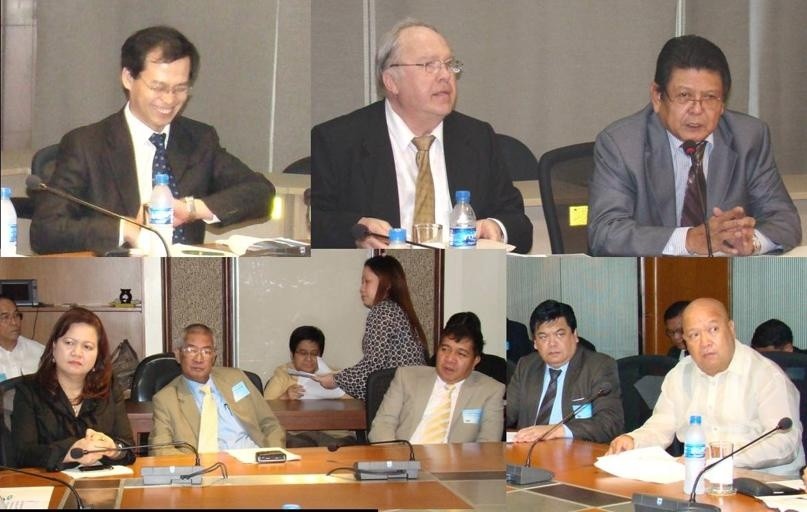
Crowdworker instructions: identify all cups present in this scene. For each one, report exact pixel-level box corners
[411,223,443,247]
[706,440,737,496]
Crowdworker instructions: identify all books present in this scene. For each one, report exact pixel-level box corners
[166,232,312,258]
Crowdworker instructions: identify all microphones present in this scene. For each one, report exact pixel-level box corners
[70,441,204,487]
[680,136,714,256]
[0,462,85,509]
[632,416,794,511]
[22,173,171,256]
[326,438,423,482]
[505,381,613,486]
[349,222,438,248]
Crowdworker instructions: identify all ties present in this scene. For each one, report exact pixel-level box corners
[149,133,184,245]
[412,136,435,244]
[198,386,218,453]
[681,141,706,226]
[536,368,562,425]
[422,384,455,444]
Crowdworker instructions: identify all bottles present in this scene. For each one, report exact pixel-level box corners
[447,189,477,247]
[1,185,18,257]
[386,228,410,249]
[148,174,174,255]
[683,415,708,494]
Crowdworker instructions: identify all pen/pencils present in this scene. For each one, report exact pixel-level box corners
[181,251,224,256]
[78,464,112,471]
[772,490,807,495]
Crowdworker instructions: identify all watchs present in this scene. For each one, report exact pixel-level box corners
[184,195,197,225]
[748,231,763,257]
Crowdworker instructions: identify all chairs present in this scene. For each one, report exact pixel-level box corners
[283,154,312,174]
[539,143,595,254]
[499,135,539,182]
[33,145,61,180]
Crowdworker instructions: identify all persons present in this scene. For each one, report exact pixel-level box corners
[602,298,807,478]
[1,298,46,383]
[508,297,627,442]
[264,325,352,403]
[367,311,507,444]
[663,298,696,358]
[10,308,136,474]
[26,22,278,257]
[304,16,533,254]
[586,32,804,257]
[145,322,288,460]
[308,255,431,401]
[750,318,802,354]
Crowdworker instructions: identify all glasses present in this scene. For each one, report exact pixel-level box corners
[390,60,463,74]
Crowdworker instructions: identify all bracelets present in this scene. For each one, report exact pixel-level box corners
[108,440,123,461]
